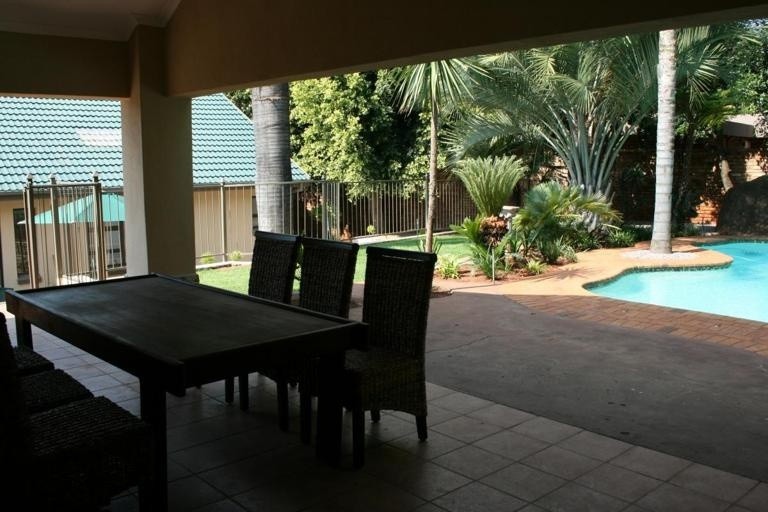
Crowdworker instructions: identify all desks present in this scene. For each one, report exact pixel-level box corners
[5,273,356,511]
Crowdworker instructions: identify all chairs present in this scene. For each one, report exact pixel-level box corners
[1,314,143,511]
[14,344,56,374]
[237,241,358,428]
[295,246,437,467]
[196,231,299,402]
[21,370,93,413]
